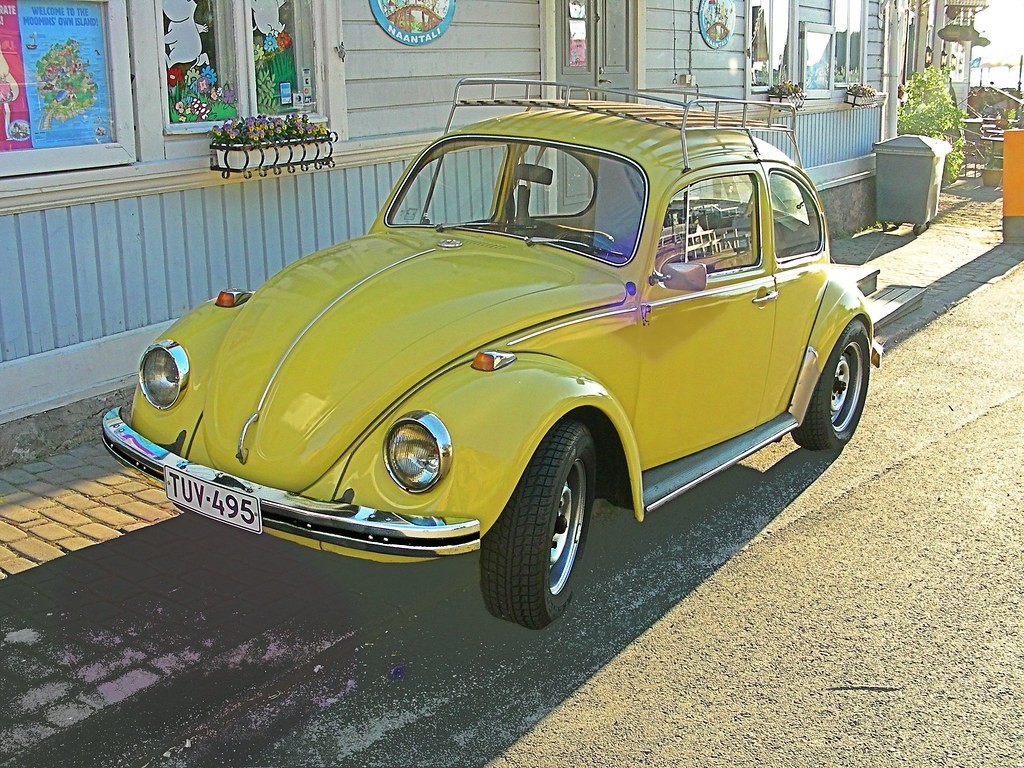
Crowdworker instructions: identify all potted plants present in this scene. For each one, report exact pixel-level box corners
[978,153,1003,188]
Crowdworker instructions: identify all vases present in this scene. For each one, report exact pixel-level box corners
[212,135,330,168]
[847,91,875,105]
[768,93,807,107]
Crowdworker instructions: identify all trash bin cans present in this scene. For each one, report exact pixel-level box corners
[872,135,954,237]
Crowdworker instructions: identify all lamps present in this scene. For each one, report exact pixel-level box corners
[925,46,934,68]
[941,50,947,67]
[950,54,957,71]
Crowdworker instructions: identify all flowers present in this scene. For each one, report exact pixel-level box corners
[848,83,878,98]
[206,112,329,148]
[767,80,803,99]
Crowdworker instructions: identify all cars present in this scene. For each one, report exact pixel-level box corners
[99,72,883,629]
